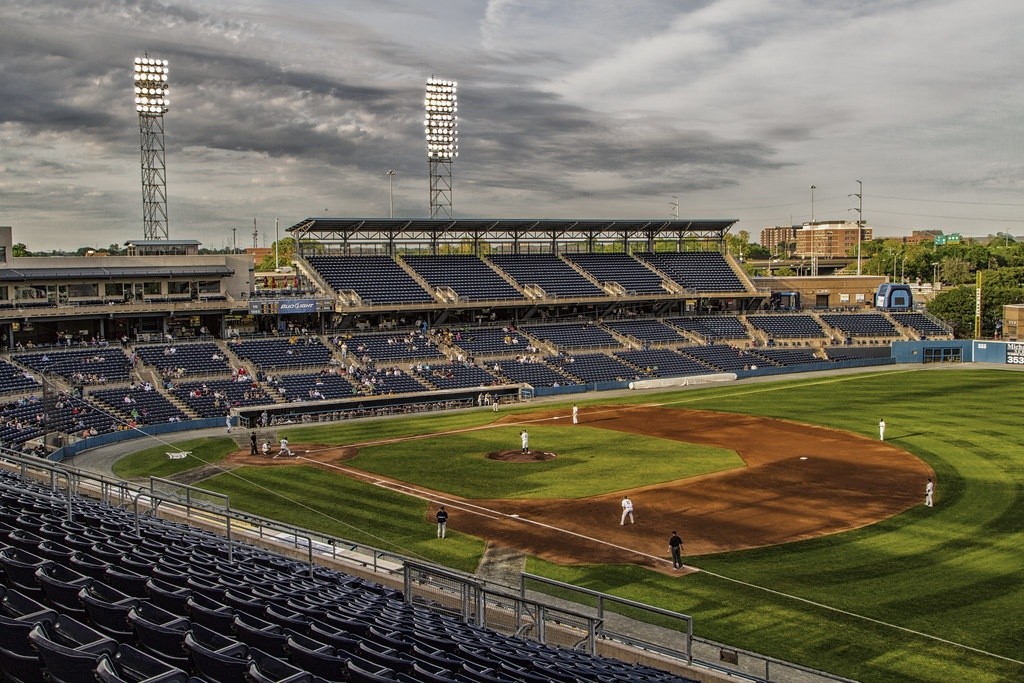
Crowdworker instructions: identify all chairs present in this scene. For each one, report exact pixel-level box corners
[0,444,693,683]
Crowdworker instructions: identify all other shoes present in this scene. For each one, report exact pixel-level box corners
[928,505,933,507]
[673,567,677,570]
[923,503,928,506]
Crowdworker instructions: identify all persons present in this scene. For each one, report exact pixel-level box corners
[262,440,271,456]
[619,496,635,526]
[696,301,775,315]
[520,430,529,454]
[0,307,659,434]
[273,437,291,459]
[250,431,259,456]
[436,505,448,539]
[263,275,298,288]
[34,426,99,457]
[879,418,886,441]
[667,531,685,570]
[682,327,1005,370]
[924,479,933,507]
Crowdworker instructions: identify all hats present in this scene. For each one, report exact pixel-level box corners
[672,531,676,534]
[441,507,444,510]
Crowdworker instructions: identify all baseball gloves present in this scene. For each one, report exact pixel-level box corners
[519,432,522,435]
